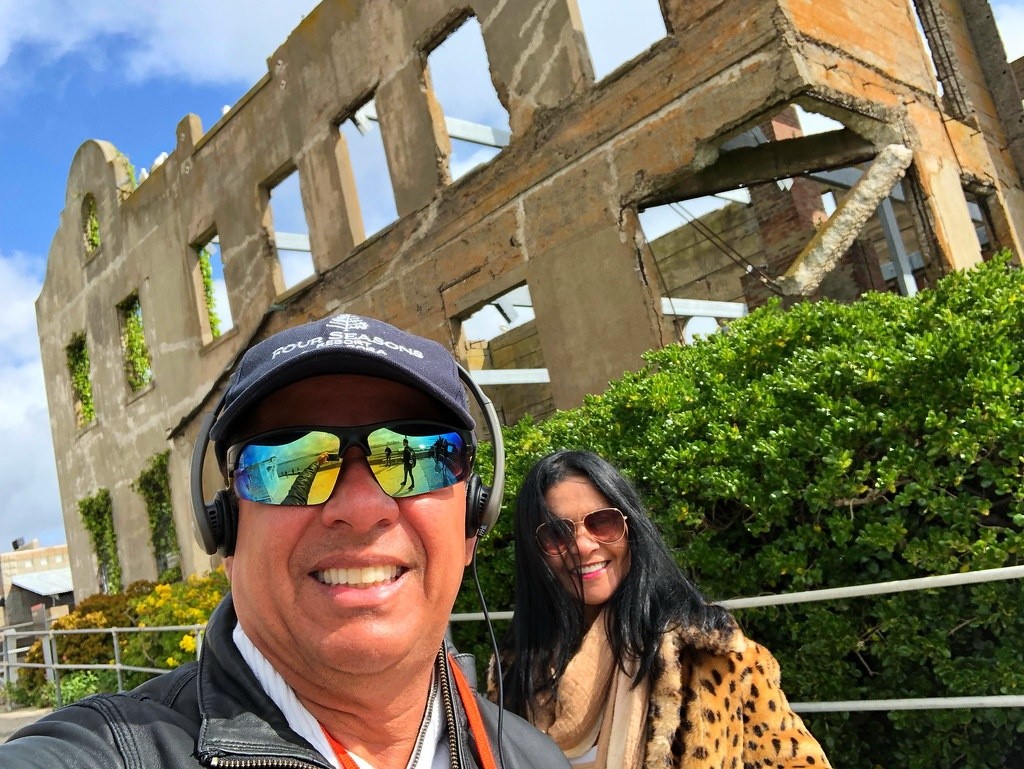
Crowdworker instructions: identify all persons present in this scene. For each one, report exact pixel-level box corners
[488,449,835,769]
[279,452,329,506]
[0,315,575,769]
[400,439,416,489]
[433,437,456,468]
[385,444,392,467]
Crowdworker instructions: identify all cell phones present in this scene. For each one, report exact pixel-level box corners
[327,453,339,461]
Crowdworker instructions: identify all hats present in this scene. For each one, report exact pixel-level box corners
[208,314,475,451]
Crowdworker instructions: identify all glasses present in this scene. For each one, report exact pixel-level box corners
[535,507,627,556]
[226,420,476,506]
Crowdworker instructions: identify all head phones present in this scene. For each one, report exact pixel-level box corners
[188,362,505,557]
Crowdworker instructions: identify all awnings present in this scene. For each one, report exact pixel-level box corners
[11,568,74,596]
